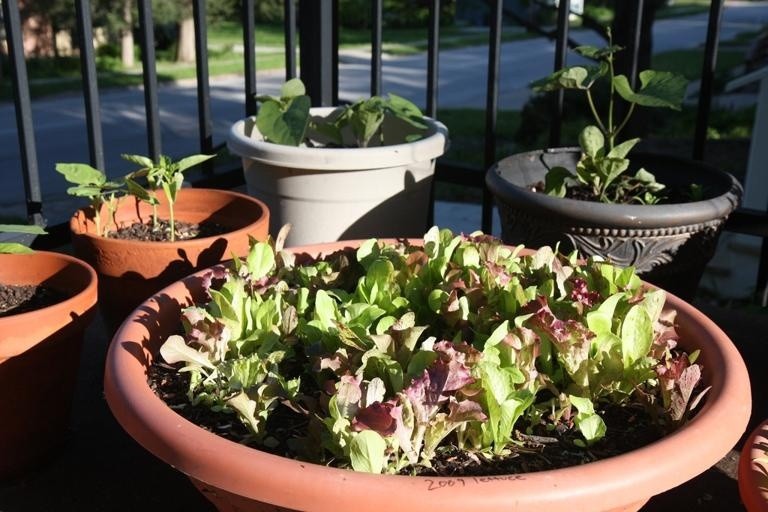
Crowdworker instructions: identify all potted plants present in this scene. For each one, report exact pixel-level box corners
[226,77,451,246]
[484,0,744,304]
[55,151,270,332]
[1,223,98,477]
[103,220,755,512]
